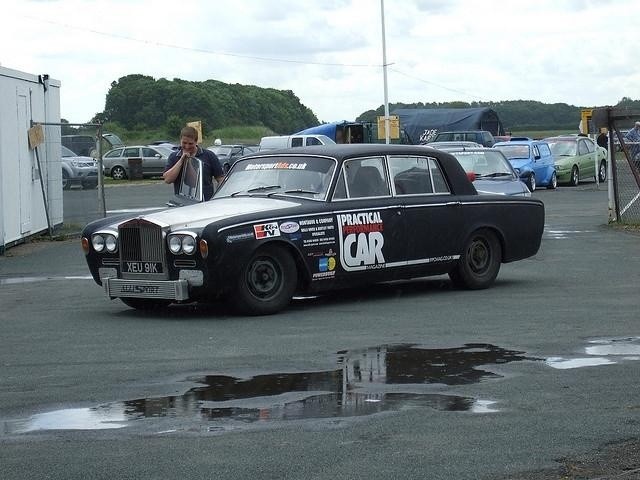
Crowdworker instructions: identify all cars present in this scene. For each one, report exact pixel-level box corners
[61,133,259,189]
[425,131,629,195]
[80,143,545,317]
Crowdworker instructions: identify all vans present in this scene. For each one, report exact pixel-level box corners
[260,134,341,149]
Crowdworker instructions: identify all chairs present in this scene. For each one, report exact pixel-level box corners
[349,165,384,196]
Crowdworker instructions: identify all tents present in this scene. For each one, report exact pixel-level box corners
[389,106,506,146]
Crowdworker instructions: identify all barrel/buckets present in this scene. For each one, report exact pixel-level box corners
[128,158,143,181]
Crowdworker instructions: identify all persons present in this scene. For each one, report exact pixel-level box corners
[162,126,225,202]
[626,121,640,176]
[596,127,609,150]
